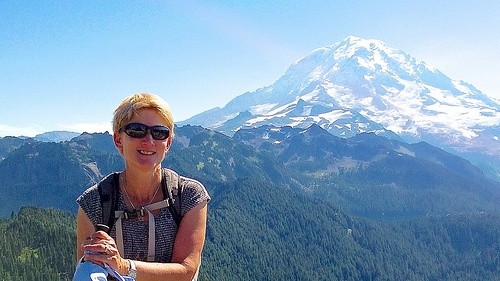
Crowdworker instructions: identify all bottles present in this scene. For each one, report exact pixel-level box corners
[73,224,110,281]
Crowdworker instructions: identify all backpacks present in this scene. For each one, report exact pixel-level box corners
[73,249,135,280]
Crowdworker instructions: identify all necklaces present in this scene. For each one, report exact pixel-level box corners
[121,173,161,221]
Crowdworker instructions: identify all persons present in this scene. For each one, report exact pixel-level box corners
[76,92,211,281]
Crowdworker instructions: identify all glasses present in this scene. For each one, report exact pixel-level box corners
[119,122,170,141]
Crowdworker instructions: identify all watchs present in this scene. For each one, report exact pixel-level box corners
[127,259,136,281]
